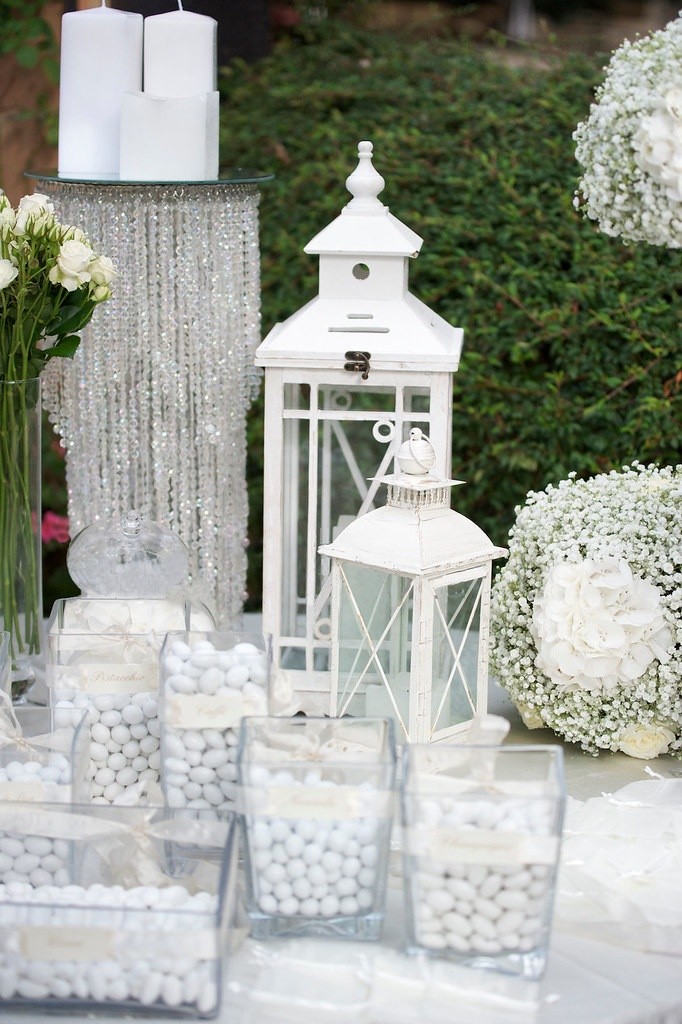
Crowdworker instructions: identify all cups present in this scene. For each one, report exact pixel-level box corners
[40,597,219,865]
[0,797,243,1024]
[1,704,94,896]
[159,630,277,881]
[397,742,565,982]
[234,714,395,940]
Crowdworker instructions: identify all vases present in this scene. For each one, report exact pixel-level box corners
[0,379,46,704]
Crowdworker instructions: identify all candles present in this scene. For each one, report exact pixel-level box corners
[143,1,217,100]
[57,1,143,181]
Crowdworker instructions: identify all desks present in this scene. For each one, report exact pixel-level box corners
[0,612,682,1022]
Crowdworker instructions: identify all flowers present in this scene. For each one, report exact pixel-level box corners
[572,7,682,250]
[480,461,682,762]
[0,184,115,675]
